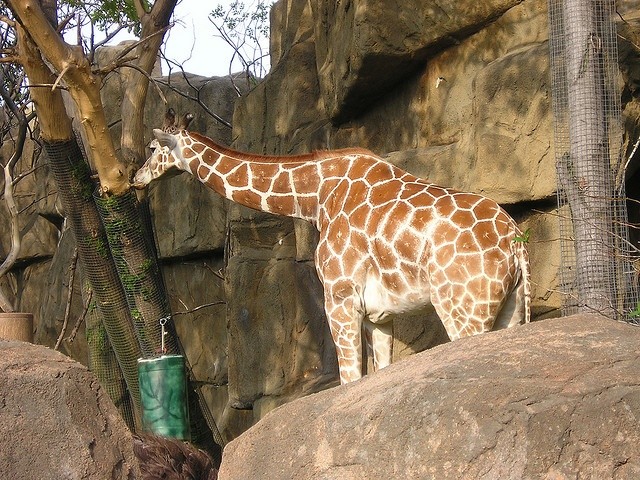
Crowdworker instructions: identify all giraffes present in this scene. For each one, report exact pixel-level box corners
[133,108,531,385]
